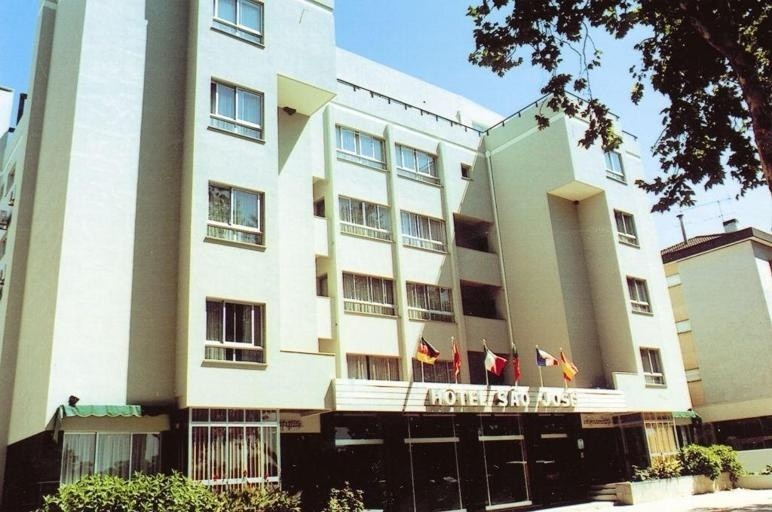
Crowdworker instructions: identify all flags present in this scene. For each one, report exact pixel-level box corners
[560,351,579,383]
[452,339,462,377]
[536,347,560,366]
[513,345,520,381]
[483,342,508,376]
[415,336,440,364]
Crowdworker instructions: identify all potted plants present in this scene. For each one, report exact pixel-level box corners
[611,441,772,506]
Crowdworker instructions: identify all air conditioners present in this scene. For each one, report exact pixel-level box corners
[0,209,8,231]
